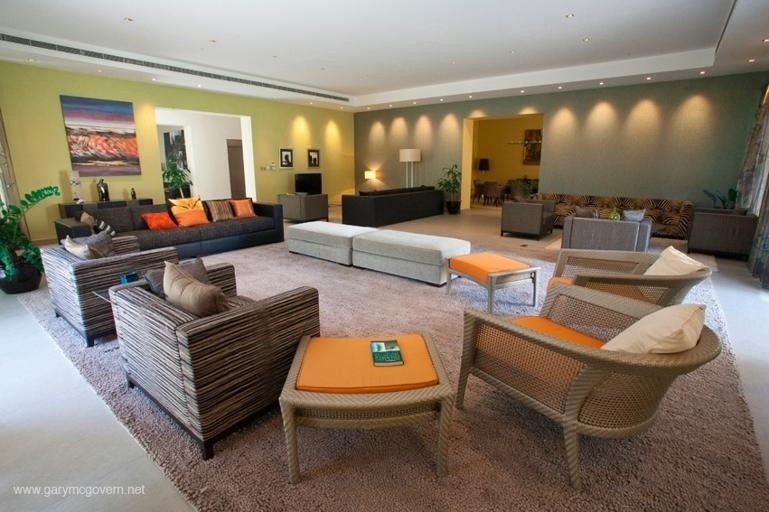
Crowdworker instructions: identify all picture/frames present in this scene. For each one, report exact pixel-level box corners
[278,147,294,169]
[306,148,320,168]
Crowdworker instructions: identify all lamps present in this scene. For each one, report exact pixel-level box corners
[398,147,422,188]
[479,157,490,199]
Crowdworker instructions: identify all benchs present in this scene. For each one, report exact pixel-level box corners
[352,229,471,284]
[287,221,381,265]
[34,165,768,493]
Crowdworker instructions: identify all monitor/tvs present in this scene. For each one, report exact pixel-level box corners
[295,173,321,195]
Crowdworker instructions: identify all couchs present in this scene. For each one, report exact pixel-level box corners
[55,198,285,259]
[342,189,444,227]
[529,192,693,236]
[501,200,557,235]
[279,192,328,221]
[688,207,757,260]
[40,236,179,347]
[108,263,320,456]
[561,213,652,252]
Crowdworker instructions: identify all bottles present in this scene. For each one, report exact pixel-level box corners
[608,207,620,220]
[131,188,136,200]
[97,179,109,201]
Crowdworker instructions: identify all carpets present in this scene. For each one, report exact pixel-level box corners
[14,239,768,511]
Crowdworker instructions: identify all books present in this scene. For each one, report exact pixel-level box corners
[369,339,404,367]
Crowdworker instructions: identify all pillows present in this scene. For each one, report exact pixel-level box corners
[163,260,232,317]
[59,235,117,259]
[599,303,705,354]
[141,199,256,229]
[622,209,644,221]
[144,257,211,301]
[643,246,710,276]
[732,207,748,216]
[73,231,116,258]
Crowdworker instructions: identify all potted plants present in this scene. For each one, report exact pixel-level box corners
[0,177,72,297]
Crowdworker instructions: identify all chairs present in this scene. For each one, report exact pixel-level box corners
[279,332,455,483]
[457,283,722,488]
[553,248,712,307]
[445,249,541,312]
